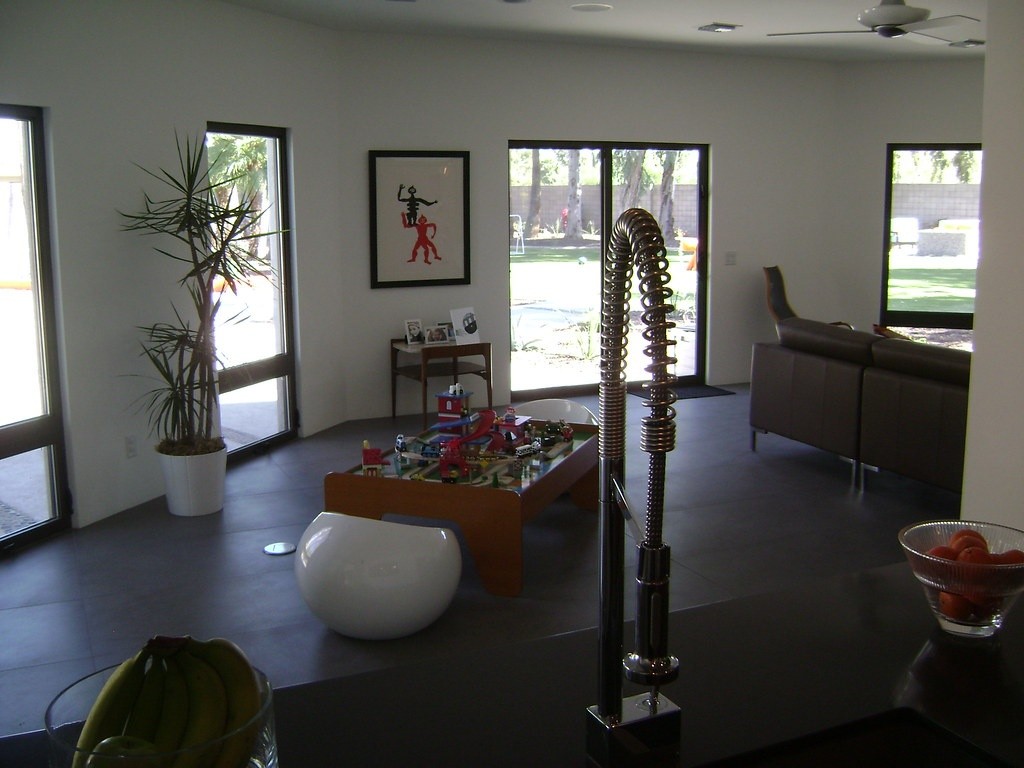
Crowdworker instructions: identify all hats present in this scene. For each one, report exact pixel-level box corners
[407,321,420,327]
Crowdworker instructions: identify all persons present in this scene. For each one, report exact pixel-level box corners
[427,328,445,341]
[406,320,426,342]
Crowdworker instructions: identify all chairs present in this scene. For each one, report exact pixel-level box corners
[748,317,972,495]
[513,399,599,425]
[294,512,460,638]
[764,266,856,330]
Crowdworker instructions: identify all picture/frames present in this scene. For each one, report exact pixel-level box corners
[368,150,470,289]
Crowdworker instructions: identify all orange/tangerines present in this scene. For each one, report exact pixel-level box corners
[924,528,1024,623]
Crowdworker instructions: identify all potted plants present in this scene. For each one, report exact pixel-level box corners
[115,125,290,517]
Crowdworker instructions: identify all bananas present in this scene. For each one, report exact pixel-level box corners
[71,634,261,768]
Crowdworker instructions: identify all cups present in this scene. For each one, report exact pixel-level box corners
[45,659,278,768]
[897,519,1024,639]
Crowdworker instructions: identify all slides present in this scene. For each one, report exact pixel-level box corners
[395,409,504,484]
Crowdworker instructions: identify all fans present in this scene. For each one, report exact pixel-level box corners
[767,0,980,45]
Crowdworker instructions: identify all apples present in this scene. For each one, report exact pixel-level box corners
[85,736,163,768]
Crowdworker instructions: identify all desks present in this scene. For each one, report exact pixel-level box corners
[324,419,600,595]
[391,339,493,431]
